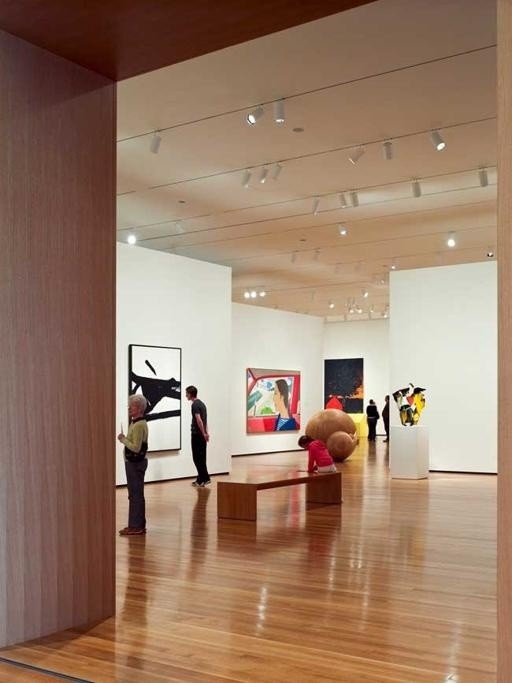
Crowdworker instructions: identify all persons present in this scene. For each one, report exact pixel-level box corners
[118,394,148,535]
[366,394,389,442]
[273,379,298,430]
[298,435,338,474]
[186,386,211,487]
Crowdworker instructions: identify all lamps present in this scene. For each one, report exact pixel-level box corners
[242,166,282,186]
[479,170,487,187]
[340,229,345,235]
[128,234,136,244]
[392,262,397,269]
[313,249,320,260]
[350,192,359,207]
[383,142,393,162]
[246,107,264,124]
[244,290,266,298]
[448,238,454,246]
[330,289,389,319]
[338,193,347,208]
[274,101,286,122]
[150,136,161,153]
[487,251,493,258]
[412,180,420,196]
[292,251,296,262]
[429,132,446,151]
[348,149,365,163]
[313,198,320,215]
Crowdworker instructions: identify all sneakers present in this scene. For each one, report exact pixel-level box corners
[118,526,147,535]
[191,481,205,487]
[204,480,211,485]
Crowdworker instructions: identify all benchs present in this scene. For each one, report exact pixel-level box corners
[216,470,343,519]
